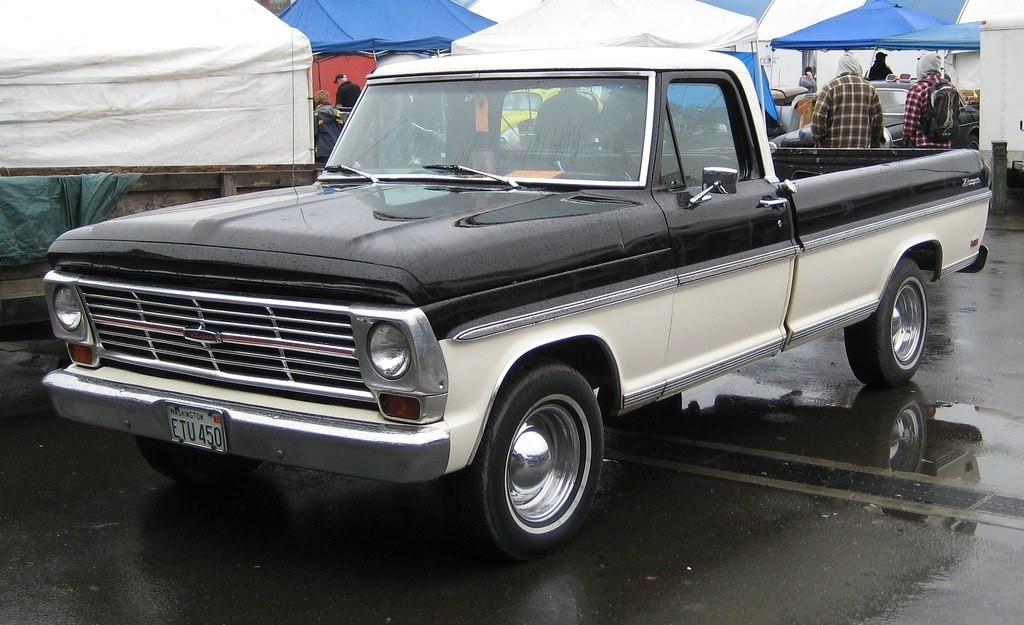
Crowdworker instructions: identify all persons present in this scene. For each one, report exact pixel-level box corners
[902,52,960,149]
[313,90,344,163]
[334,74,361,111]
[869,52,892,80]
[811,53,886,148]
[535,84,645,150]
[800,67,816,93]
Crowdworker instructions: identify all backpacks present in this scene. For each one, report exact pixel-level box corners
[915,78,960,142]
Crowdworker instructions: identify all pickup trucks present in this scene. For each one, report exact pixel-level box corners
[40,41,995,562]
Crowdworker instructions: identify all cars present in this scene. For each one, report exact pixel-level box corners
[766,73,979,148]
[499,88,603,147]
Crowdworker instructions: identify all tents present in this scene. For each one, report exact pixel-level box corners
[280,0,981,133]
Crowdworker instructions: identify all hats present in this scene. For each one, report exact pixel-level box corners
[804,67,813,72]
[334,73,347,83]
[876,52,887,59]
